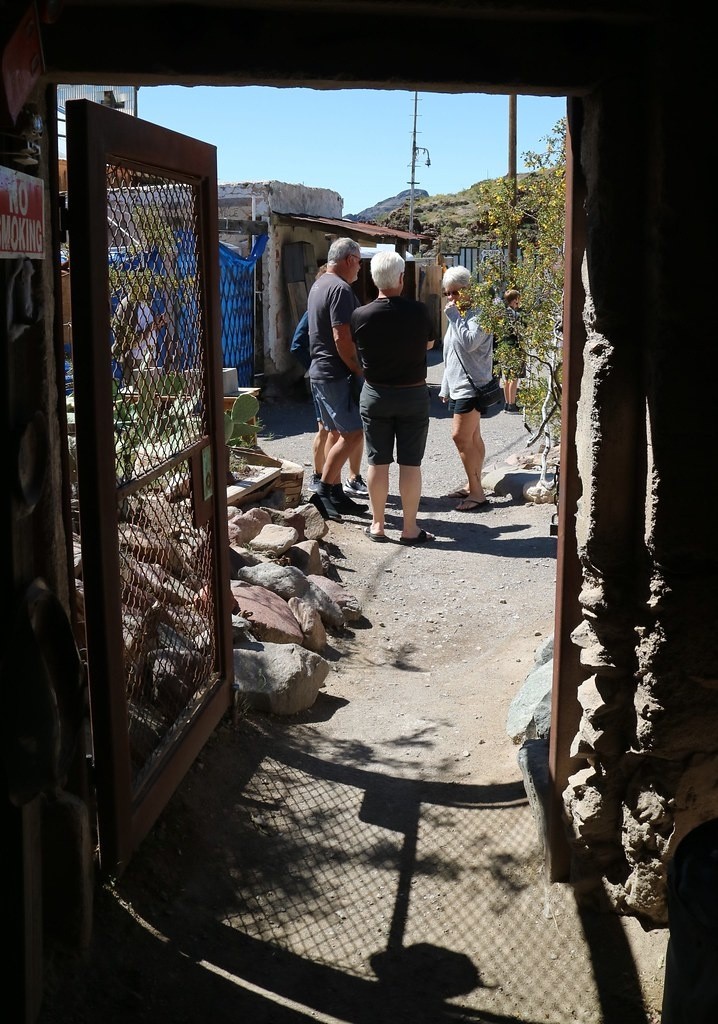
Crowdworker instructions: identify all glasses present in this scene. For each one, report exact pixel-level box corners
[346,254,364,266]
[443,287,465,296]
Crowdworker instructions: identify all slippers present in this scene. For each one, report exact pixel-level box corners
[399,530,436,544]
[456,499,489,511]
[449,491,468,498]
[362,525,387,542]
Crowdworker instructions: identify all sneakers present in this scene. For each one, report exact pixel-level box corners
[308,470,322,492]
[343,475,369,494]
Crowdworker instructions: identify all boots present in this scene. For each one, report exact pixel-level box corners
[309,482,341,522]
[329,483,368,514]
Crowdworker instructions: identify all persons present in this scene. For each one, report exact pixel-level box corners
[113,265,170,371]
[290,263,368,496]
[438,265,494,511]
[492,290,532,414]
[349,251,436,544]
[304,237,369,523]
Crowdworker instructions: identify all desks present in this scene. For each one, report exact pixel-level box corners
[116,385,261,446]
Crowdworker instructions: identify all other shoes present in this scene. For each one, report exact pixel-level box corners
[508,404,520,412]
[505,403,508,411]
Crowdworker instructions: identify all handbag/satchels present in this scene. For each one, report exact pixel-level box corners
[475,380,505,418]
